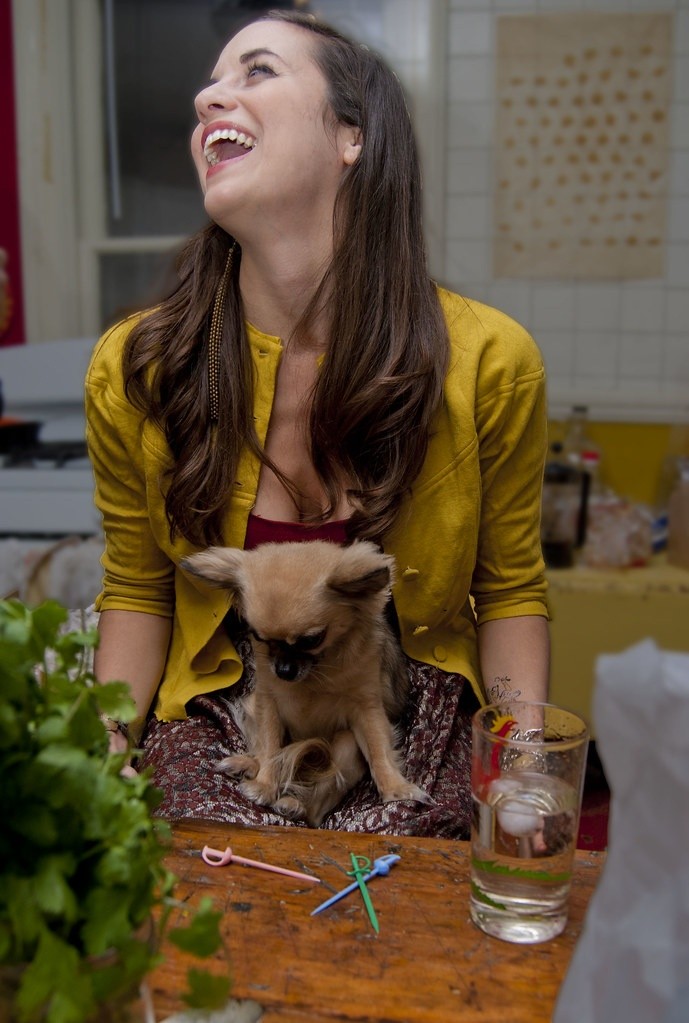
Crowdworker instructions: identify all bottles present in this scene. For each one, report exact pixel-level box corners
[583,450,617,550]
[564,406,587,470]
[668,461,689,568]
[551,443,562,466]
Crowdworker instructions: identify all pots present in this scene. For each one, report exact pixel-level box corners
[0,420,42,448]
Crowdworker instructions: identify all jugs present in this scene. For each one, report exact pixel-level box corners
[540,466,591,569]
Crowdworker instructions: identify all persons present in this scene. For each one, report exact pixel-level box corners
[86,8,552,854]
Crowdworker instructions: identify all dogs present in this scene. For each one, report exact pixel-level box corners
[185,541,437,823]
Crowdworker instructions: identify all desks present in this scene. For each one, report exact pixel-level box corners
[121,826,608,1023]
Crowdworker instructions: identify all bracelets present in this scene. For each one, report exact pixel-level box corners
[501,727,547,775]
[100,712,138,752]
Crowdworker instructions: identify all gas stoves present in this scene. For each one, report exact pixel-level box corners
[0,340,102,533]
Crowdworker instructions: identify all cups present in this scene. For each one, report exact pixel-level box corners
[471,701,590,944]
